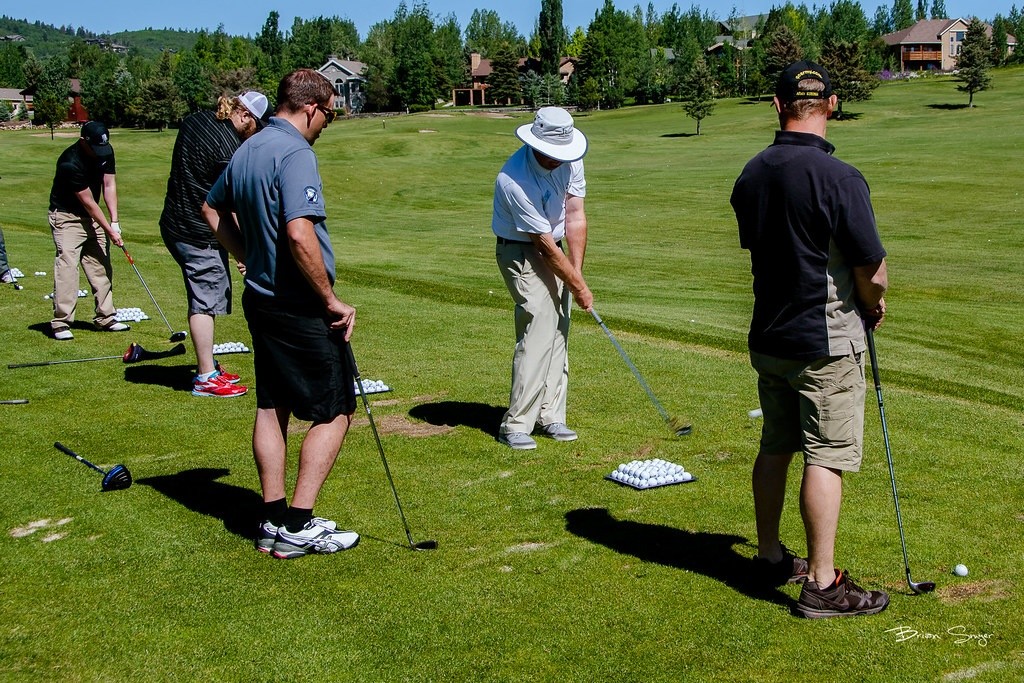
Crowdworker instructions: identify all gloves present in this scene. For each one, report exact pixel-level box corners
[111,222,121,235]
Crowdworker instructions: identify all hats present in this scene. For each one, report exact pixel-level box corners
[514,106,589,163]
[770,60,832,107]
[81,121,112,156]
[238,92,274,128]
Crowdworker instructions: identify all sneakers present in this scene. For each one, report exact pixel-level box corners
[107,321,130,331]
[1,275,18,284]
[273,519,360,559]
[256,515,337,553]
[52,326,74,340]
[533,423,578,441]
[795,569,891,619]
[191,371,248,397]
[752,540,808,585]
[191,360,240,384]
[498,432,537,450]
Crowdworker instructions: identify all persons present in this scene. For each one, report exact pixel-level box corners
[0,227,18,284]
[48,122,132,340]
[492,106,594,450]
[159,92,273,397]
[202,70,361,560]
[730,62,890,621]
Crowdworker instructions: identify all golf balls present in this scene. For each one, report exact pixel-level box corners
[955,564,968,576]
[181,330,188,336]
[354,378,390,394]
[34,271,47,276]
[10,267,24,278]
[45,295,49,299]
[19,286,23,290]
[50,289,89,298]
[611,458,692,488]
[747,409,762,420]
[114,307,148,323]
[213,341,249,356]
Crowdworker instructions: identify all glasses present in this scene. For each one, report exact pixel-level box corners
[304,101,338,123]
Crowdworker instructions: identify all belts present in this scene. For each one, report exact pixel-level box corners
[497,236,561,244]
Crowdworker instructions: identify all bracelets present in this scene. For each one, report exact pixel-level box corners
[111,220,119,223]
[863,304,880,312]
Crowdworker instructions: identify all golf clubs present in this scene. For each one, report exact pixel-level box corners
[590,307,694,437]
[52,441,132,492]
[8,355,123,369]
[865,315,937,595]
[343,327,439,551]
[1,243,20,290]
[121,245,186,342]
